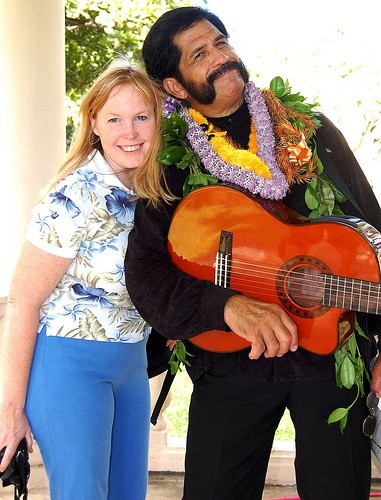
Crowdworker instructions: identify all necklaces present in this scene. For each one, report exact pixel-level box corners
[179,81,290,201]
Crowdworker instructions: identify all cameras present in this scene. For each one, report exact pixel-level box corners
[0,437,30,494]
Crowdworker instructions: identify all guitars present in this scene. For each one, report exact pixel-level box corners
[164,183,381,356]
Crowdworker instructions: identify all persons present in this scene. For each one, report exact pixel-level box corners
[122,7,381,500]
[0,51,179,500]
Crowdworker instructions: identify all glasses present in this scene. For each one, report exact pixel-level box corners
[363,392,381,450]
